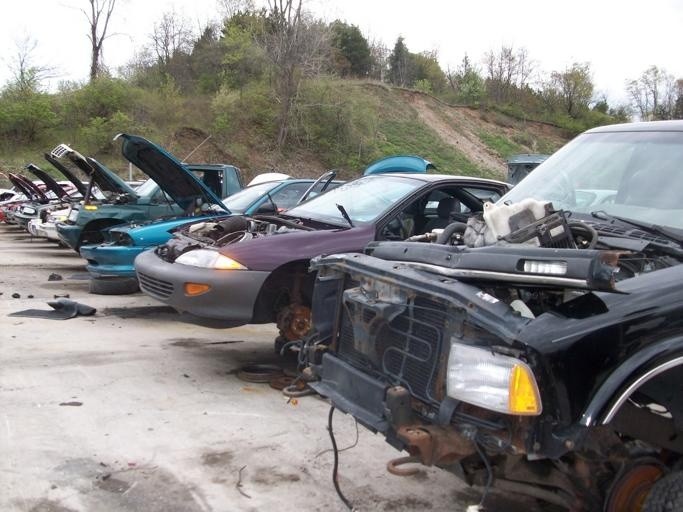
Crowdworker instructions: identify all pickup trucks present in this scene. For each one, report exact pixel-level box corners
[55,157,245,252]
[301,119,683,512]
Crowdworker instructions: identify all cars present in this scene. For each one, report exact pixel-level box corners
[79,132,347,278]
[134,171,515,343]
[0,143,106,240]
